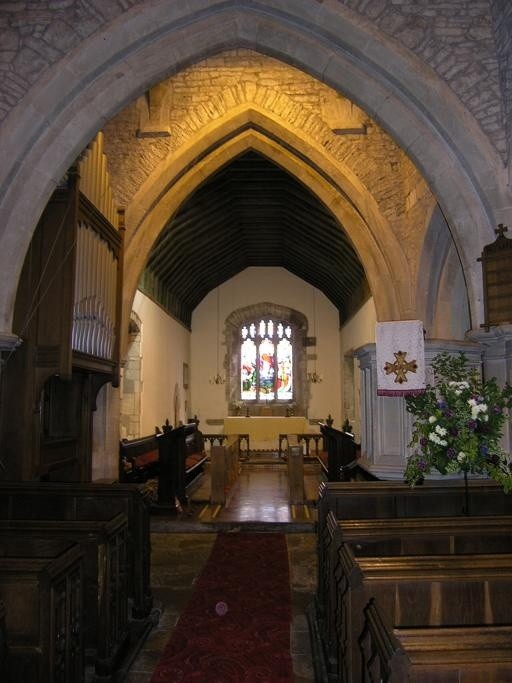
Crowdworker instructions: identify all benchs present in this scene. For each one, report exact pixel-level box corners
[0,478,163,683]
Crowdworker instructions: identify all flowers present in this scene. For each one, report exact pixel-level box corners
[403,350,511,497]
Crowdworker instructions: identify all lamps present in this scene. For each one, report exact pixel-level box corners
[376,321,426,396]
